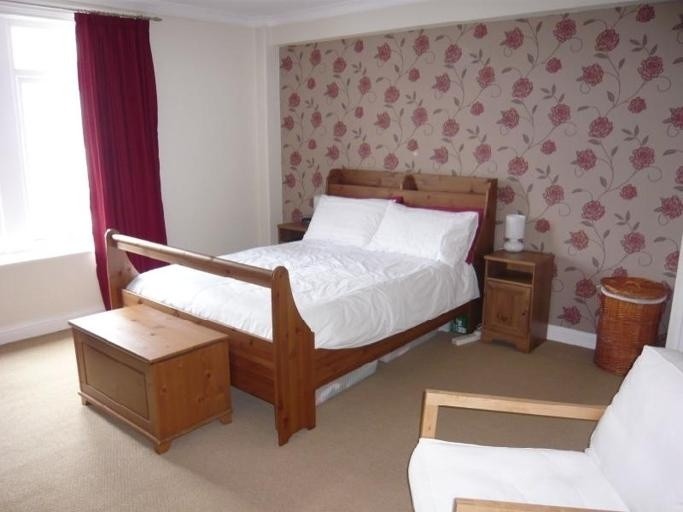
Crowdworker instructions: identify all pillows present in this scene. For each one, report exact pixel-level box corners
[370,202,478,265]
[303,195,392,249]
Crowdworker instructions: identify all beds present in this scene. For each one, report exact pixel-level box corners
[105,165,496,446]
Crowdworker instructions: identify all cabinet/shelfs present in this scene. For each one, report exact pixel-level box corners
[67,303,233,455]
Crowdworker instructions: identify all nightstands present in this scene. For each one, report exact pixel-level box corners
[276,222,306,244]
[479,248,556,353]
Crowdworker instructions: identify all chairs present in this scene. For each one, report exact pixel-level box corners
[407,346,683,512]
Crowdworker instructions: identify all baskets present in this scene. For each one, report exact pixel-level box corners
[594,275,673,377]
